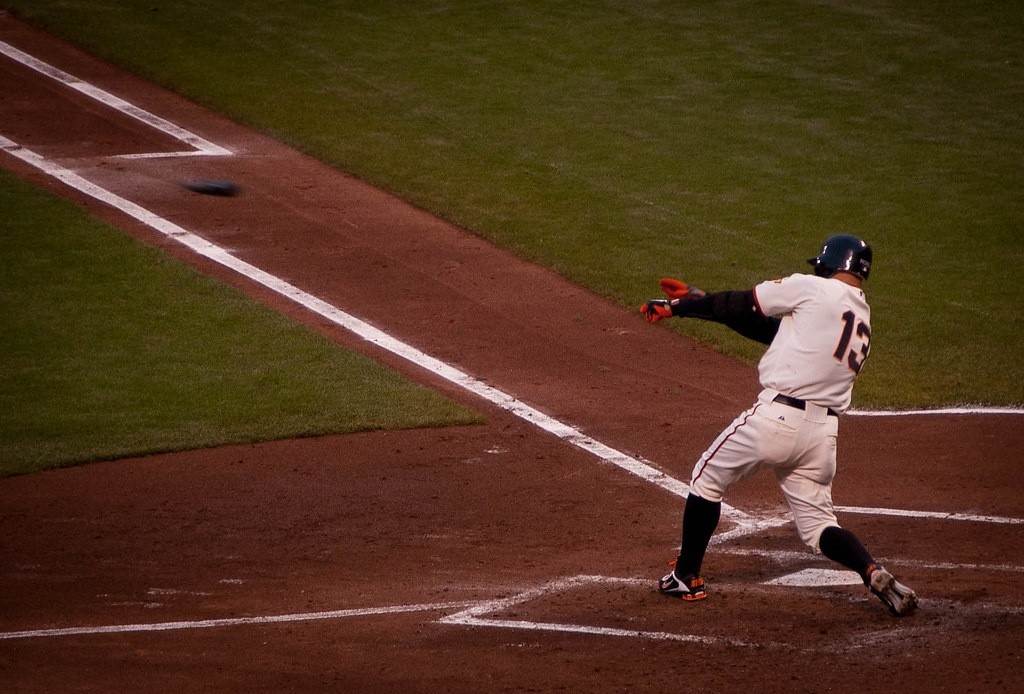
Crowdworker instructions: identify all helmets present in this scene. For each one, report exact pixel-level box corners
[807,234,872,282]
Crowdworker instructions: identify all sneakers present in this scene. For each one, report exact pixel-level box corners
[656,570,706,600]
[863,564,920,615]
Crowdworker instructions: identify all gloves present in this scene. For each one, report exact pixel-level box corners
[660,279,706,301]
[639,299,673,324]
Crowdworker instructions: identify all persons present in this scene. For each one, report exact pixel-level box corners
[639,235,919,611]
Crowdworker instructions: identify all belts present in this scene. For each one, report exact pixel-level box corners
[773,394,838,417]
